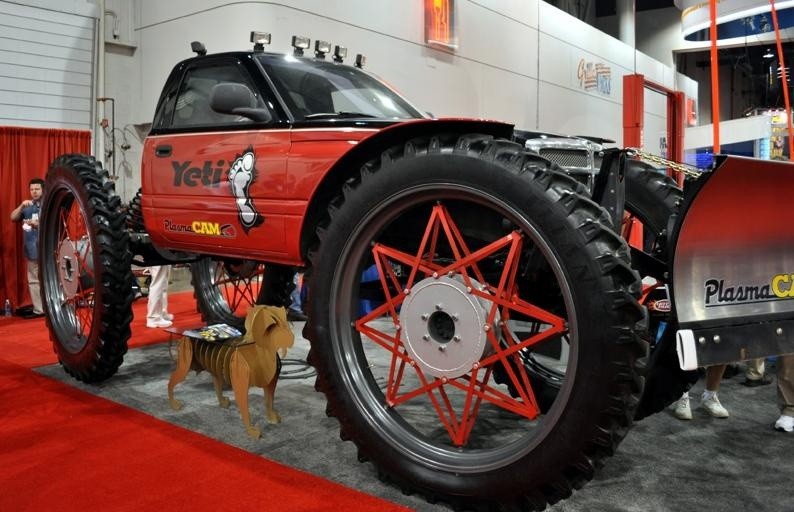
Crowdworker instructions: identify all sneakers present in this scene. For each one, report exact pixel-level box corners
[774,415,794,431]
[674,396,692,420]
[698,390,729,418]
[146,313,174,328]
[287,312,308,321]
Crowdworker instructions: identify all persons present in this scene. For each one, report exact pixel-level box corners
[147,265,175,328]
[10,178,46,320]
[774,356,794,434]
[674,363,730,420]
[724,357,772,388]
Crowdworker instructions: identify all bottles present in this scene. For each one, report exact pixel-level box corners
[32,203,39,222]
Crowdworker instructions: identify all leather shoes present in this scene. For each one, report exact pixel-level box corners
[745,374,774,387]
[24,312,45,318]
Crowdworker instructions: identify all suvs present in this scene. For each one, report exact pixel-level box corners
[36,32,794,512]
